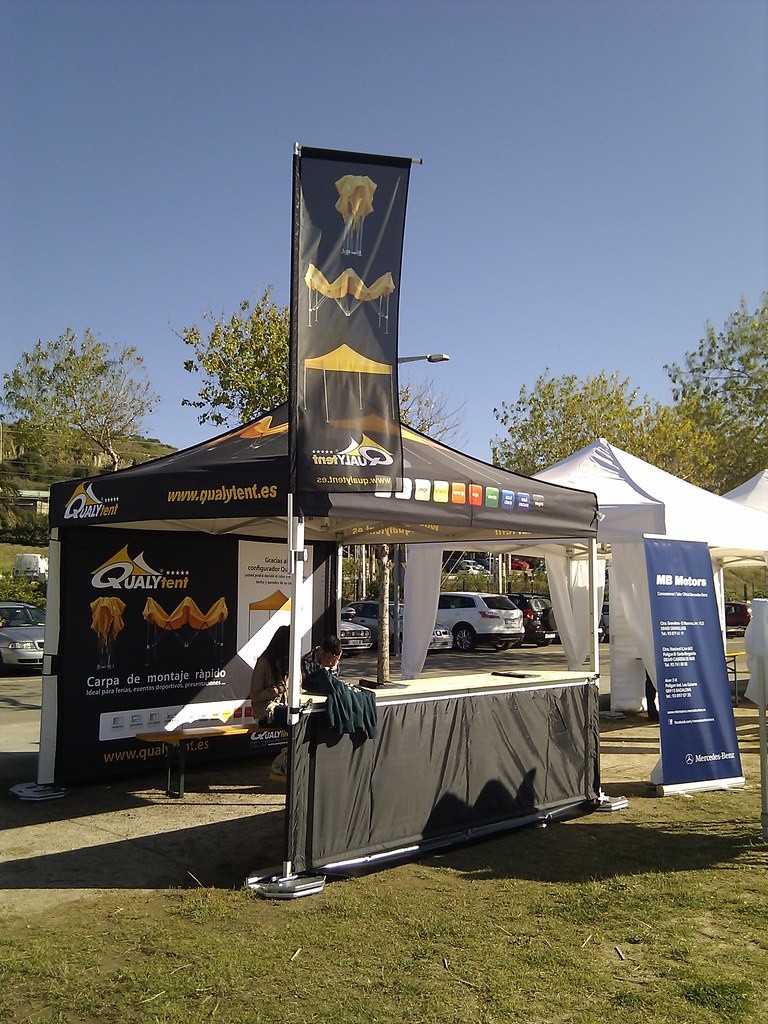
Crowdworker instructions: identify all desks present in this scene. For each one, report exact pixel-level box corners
[636,651,746,708]
[300,669,599,878]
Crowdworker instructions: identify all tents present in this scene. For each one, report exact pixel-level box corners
[9,373,629,899]
[400,436,768,838]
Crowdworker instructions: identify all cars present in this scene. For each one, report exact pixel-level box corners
[596,602,613,643]
[340,620,376,657]
[443,555,546,575]
[0,601,47,674]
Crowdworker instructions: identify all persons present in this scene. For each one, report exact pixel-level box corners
[299,636,343,682]
[250,625,301,730]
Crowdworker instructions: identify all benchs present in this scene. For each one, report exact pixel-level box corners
[141,725,271,798]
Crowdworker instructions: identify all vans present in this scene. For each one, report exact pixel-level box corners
[12,552,48,585]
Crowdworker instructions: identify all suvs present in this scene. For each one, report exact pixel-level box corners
[342,598,454,657]
[499,592,559,646]
[435,591,526,653]
[725,601,752,638]
